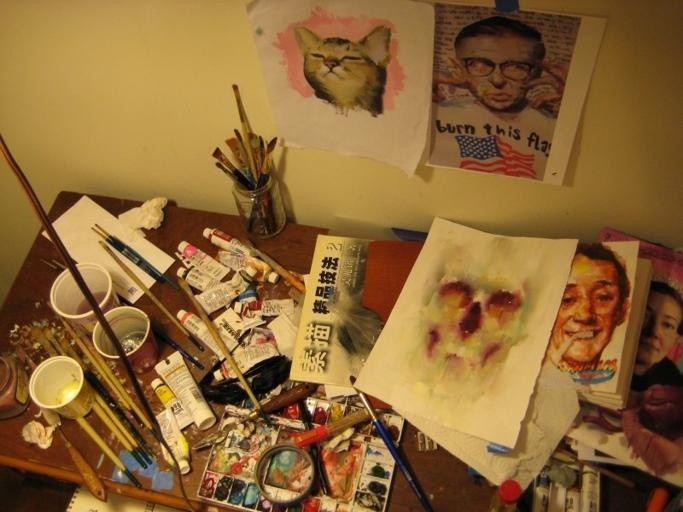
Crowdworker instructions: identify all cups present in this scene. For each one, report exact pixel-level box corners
[26,356,95,420]
[231,175,288,239]
[51,261,161,374]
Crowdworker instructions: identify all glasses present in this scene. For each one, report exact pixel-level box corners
[462,56,533,81]
[199,331,291,403]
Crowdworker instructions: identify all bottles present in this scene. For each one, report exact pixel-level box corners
[0,356,30,419]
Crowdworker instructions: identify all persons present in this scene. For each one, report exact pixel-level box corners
[630,280,683,392]
[431,17,567,182]
[553,242,631,381]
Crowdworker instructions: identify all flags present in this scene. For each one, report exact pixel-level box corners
[455,135,537,179]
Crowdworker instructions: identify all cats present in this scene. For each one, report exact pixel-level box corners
[293,25,391,117]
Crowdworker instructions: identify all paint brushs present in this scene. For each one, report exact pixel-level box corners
[206,85,287,187]
[349,375,438,511]
[178,277,274,427]
[4,322,165,488]
[84,223,205,355]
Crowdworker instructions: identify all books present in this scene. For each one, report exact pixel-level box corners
[543,240,651,411]
[532,460,600,512]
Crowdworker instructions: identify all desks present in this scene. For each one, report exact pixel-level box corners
[0,190,682,510]
